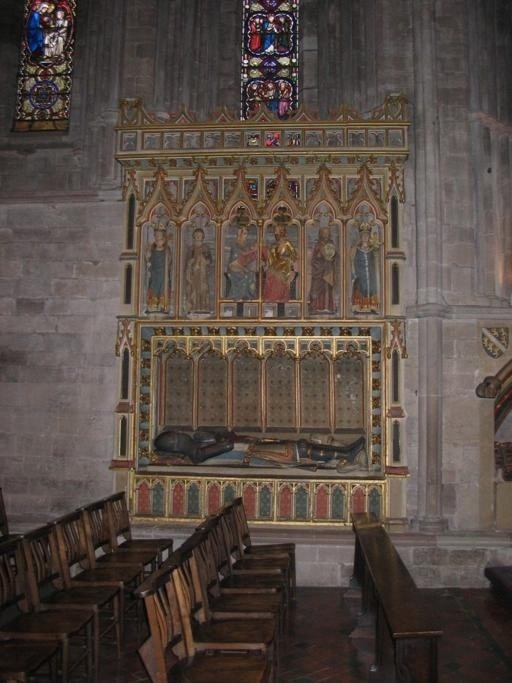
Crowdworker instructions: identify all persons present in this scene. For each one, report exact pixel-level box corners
[185,228,212,312]
[153,427,366,467]
[348,220,382,315]
[309,227,337,314]
[146,230,171,315]
[262,226,299,302]
[225,227,257,300]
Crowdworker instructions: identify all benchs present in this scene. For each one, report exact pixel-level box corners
[350,512,444,683]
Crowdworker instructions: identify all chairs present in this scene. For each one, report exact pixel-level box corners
[133,497,295,683]
[3,493,174,683]
